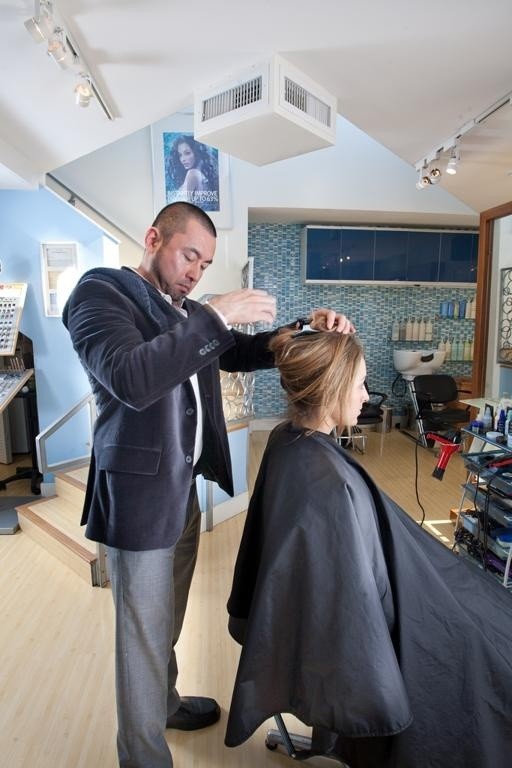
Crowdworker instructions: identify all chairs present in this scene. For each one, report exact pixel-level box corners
[337,380,392,454]
[399,373,473,451]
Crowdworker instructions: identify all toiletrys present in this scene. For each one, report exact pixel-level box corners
[392,315,432,340]
[441,293,476,318]
[437,337,474,360]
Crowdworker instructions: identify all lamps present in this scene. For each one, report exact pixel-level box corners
[18,1,102,117]
[408,135,465,195]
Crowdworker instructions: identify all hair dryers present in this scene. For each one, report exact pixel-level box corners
[424,430,462,481]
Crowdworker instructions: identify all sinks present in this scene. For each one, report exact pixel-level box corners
[392,349,446,381]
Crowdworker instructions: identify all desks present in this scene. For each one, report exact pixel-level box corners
[457,396,501,458]
[1,368,44,495]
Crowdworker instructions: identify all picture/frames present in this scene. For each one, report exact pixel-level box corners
[149,110,237,232]
[36,237,84,320]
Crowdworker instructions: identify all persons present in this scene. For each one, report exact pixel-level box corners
[62,201,358,768]
[168,135,219,210]
[267,321,370,446]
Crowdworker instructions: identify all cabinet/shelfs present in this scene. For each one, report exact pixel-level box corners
[371,227,440,287]
[296,224,374,288]
[389,315,476,365]
[436,230,479,289]
[448,421,512,593]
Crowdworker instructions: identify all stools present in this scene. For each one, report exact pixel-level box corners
[262,705,351,768]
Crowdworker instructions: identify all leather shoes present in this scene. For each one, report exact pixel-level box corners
[166,695,221,732]
